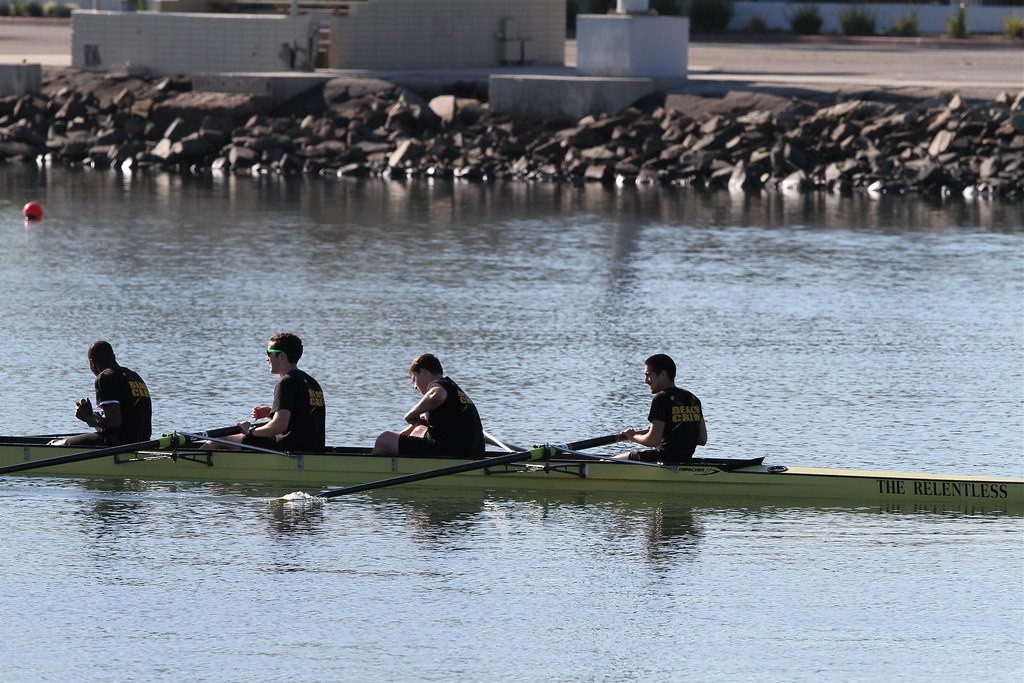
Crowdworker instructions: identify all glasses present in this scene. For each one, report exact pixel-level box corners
[266,349,287,357]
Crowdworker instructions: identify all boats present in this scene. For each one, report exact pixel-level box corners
[1,432,1024,513]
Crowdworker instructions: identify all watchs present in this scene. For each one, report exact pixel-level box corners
[248,426,257,436]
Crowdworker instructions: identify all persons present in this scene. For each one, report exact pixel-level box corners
[610,354,708,463]
[199,332,325,450]
[372,353,485,459]
[46,341,151,445]
